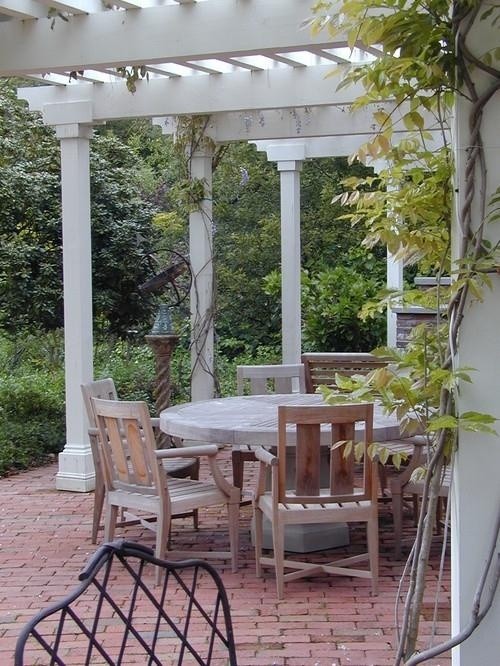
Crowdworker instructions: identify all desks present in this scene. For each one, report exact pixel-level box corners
[157,390,437,555]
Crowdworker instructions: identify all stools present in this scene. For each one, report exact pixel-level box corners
[395,430,456,556]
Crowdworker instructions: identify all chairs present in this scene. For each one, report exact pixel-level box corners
[297,351,425,530]
[233,363,312,503]
[15,538,238,666]
[90,398,242,587]
[77,378,203,551]
[251,405,383,600]
